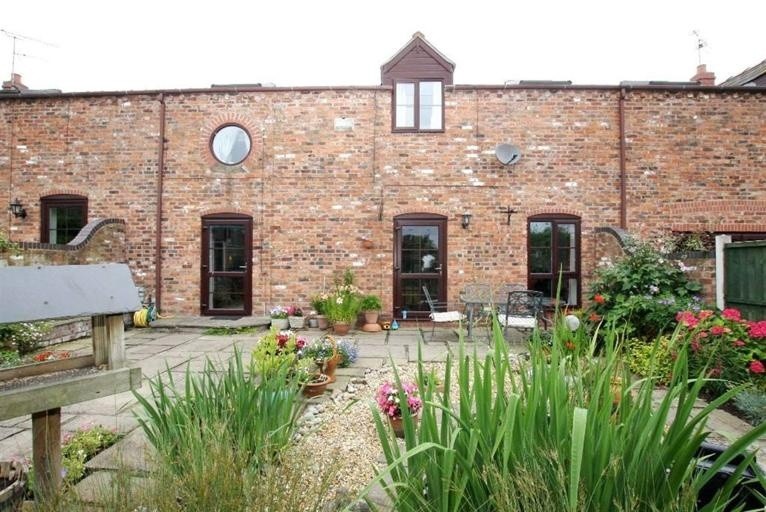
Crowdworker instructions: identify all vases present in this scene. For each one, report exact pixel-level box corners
[389,413,419,438]
[297,373,330,398]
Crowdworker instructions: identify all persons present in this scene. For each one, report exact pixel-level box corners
[422,255,437,299]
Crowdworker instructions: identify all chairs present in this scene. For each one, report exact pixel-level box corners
[422,282,566,342]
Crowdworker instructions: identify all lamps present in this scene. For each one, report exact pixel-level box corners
[462,211,473,229]
[9,197,27,220]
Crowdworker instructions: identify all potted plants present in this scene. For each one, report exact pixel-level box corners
[360,293,383,332]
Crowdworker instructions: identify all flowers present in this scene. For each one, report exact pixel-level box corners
[254,267,364,385]
[373,380,423,420]
